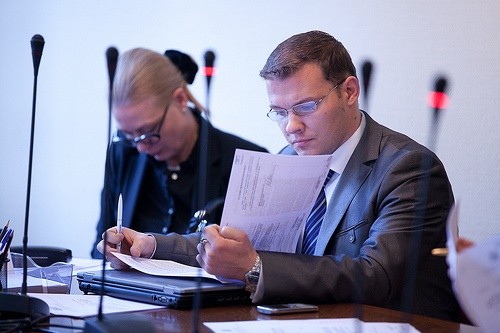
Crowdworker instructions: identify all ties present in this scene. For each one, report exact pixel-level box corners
[302,169,335,255]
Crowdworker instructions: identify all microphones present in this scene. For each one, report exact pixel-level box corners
[192,49,217,333]
[83,47,154,333]
[353,59,373,333]
[21,34,46,294]
[401,76,449,333]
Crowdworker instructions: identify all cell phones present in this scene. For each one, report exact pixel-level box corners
[256,303,318,315]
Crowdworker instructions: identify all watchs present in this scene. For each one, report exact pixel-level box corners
[245,253,262,294]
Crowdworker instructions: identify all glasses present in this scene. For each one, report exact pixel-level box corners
[267,75,357,122]
[117,87,178,147]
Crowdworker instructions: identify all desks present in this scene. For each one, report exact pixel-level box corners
[0,258,484,333]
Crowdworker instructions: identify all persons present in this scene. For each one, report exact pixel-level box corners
[97,30,476,326]
[90,46,271,259]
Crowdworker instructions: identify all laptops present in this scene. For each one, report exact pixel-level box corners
[77,267,254,307]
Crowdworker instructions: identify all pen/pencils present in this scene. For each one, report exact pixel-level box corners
[0,219,14,272]
[116,192,124,253]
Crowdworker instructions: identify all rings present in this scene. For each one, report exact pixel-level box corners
[200,239,207,250]
